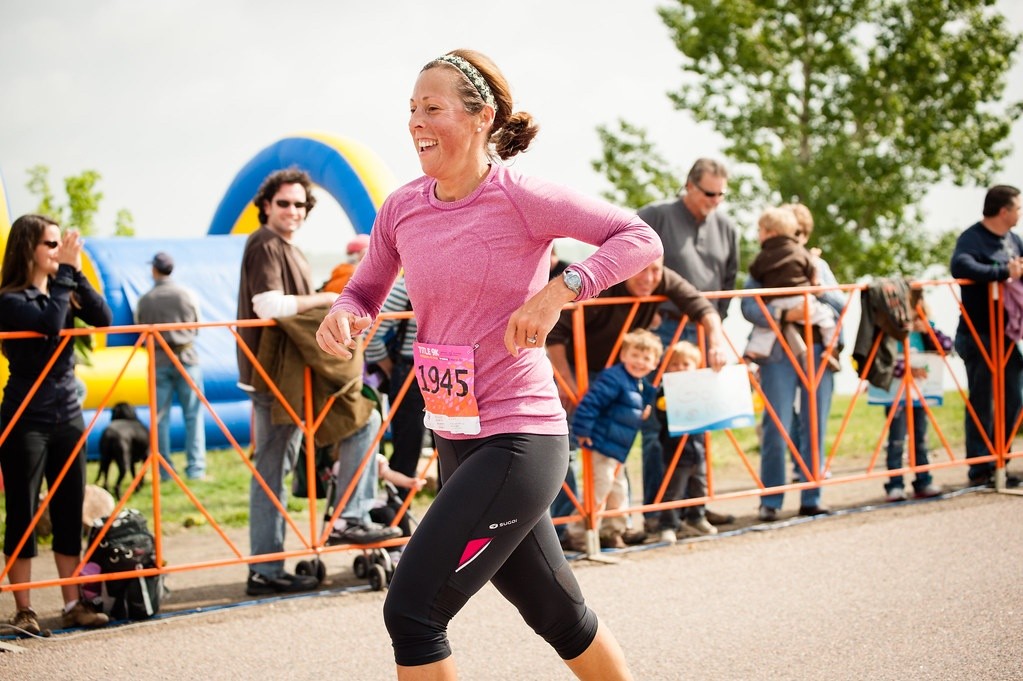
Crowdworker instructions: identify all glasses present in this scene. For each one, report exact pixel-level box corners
[275,200,308,209]
[695,183,726,198]
[795,230,805,237]
[39,240,59,249]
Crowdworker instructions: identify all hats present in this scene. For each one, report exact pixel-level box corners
[346,234,370,253]
[147,252,174,273]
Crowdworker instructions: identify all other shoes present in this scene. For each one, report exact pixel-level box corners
[758,504,780,520]
[686,517,718,535]
[739,356,760,390]
[820,347,841,371]
[644,517,660,532]
[792,473,801,482]
[885,487,908,501]
[799,504,832,516]
[705,506,735,525]
[659,527,677,544]
[969,470,1022,488]
[600,531,625,548]
[914,483,942,498]
[190,474,214,482]
[819,469,832,478]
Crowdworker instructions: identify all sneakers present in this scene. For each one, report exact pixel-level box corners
[328,518,403,545]
[14,608,52,638]
[60,601,109,629]
[245,569,319,596]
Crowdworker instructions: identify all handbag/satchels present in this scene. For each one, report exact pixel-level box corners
[367,301,413,376]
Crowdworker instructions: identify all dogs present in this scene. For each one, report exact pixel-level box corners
[93,401,150,502]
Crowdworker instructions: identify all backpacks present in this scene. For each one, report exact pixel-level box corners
[79,507,171,621]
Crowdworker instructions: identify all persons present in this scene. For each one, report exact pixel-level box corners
[138,251,215,481]
[314,48,663,681]
[950,186,1023,489]
[0,216,112,637]
[234,167,850,598]
[882,284,952,501]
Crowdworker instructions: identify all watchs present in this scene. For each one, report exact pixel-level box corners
[562,270,581,294]
